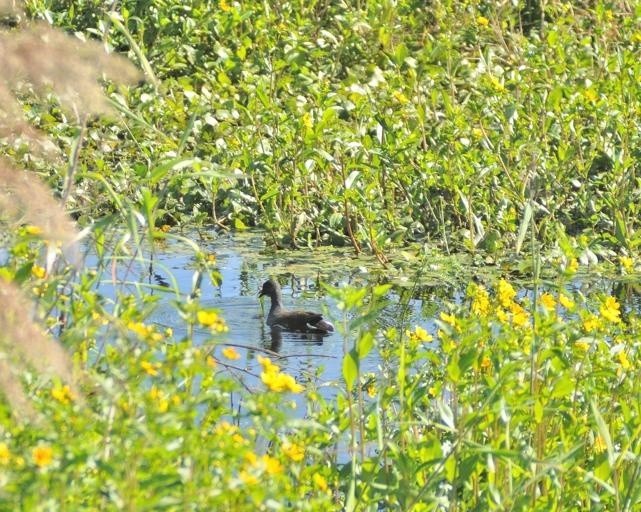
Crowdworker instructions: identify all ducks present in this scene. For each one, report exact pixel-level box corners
[257,279,334,333]
